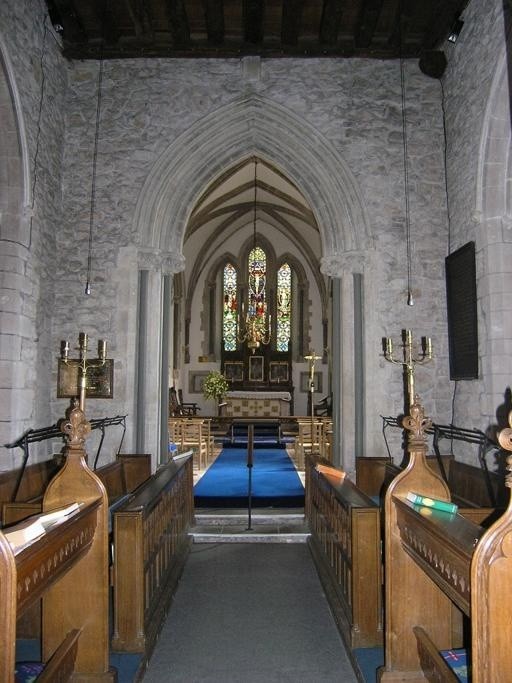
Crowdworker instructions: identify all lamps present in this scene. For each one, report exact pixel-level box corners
[235,157,275,356]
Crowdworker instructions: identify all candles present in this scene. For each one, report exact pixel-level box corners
[428,336,433,358]
[102,339,108,358]
[407,329,413,345]
[82,333,89,346]
[387,338,394,354]
[64,340,70,356]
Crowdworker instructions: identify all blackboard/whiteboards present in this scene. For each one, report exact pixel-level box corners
[445,240,478,381]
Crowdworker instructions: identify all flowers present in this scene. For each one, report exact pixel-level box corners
[201,368,229,401]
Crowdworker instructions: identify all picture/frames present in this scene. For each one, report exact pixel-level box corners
[249,355,266,382]
[224,360,244,382]
[269,362,289,383]
[57,358,114,400]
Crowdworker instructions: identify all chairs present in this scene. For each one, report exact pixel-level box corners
[292,417,333,470]
[168,416,216,471]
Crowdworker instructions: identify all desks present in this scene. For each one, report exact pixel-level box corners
[220,391,292,418]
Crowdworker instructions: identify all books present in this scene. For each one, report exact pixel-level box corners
[0,517,47,553]
[440,648,471,683]
[172,447,194,460]
[21,501,81,523]
[314,464,346,480]
[405,491,458,514]
